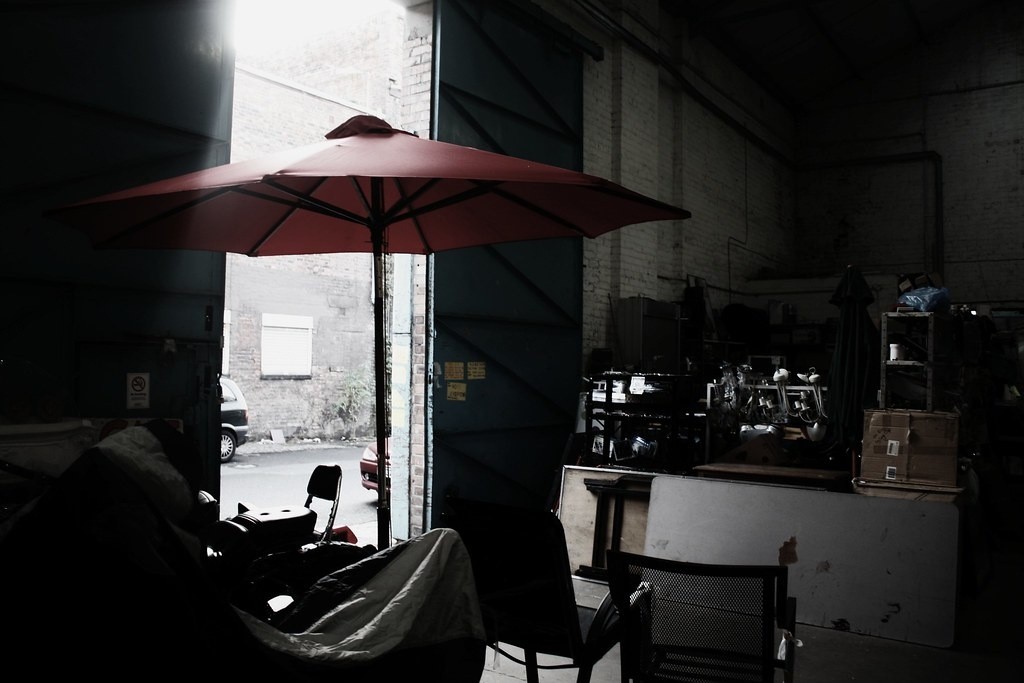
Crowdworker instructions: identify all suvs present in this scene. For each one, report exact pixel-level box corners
[218,374,251,463]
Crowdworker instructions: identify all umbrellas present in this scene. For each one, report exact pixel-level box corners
[824,264,882,477]
[41,114,693,551]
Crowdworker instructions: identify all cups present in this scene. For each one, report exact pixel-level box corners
[889,343,905,359]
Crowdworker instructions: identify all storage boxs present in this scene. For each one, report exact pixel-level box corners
[861,409,958,488]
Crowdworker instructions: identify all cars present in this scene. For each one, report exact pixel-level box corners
[359,437,390,492]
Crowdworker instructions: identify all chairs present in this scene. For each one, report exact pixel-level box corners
[452,470,625,683]
[599,545,799,682]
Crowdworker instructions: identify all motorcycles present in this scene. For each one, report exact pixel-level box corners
[0,416,455,682]
